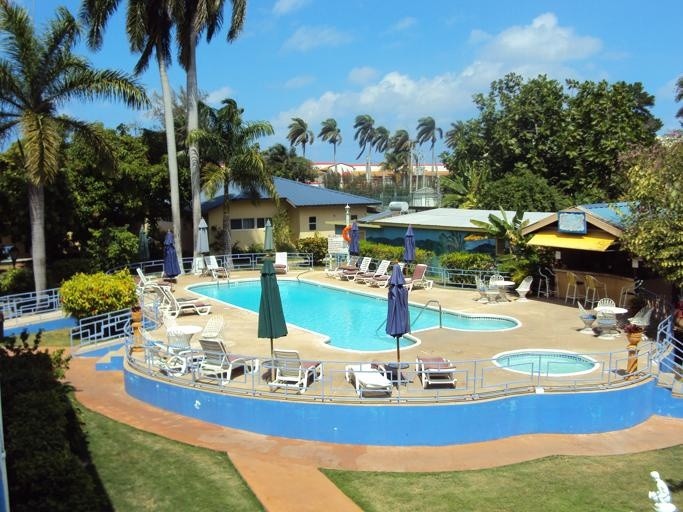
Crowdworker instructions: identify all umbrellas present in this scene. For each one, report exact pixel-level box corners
[162,228,181,288]
[385,258,410,364]
[136,224,149,268]
[263,218,274,256]
[348,220,359,262]
[403,224,414,266]
[196,217,209,267]
[257,254,287,360]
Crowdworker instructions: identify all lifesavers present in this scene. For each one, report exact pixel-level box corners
[342,225,352,241]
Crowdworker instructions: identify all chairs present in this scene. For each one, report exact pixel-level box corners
[206,255,234,284]
[271,248,291,275]
[324,250,435,294]
[539,268,647,333]
[476,269,536,308]
